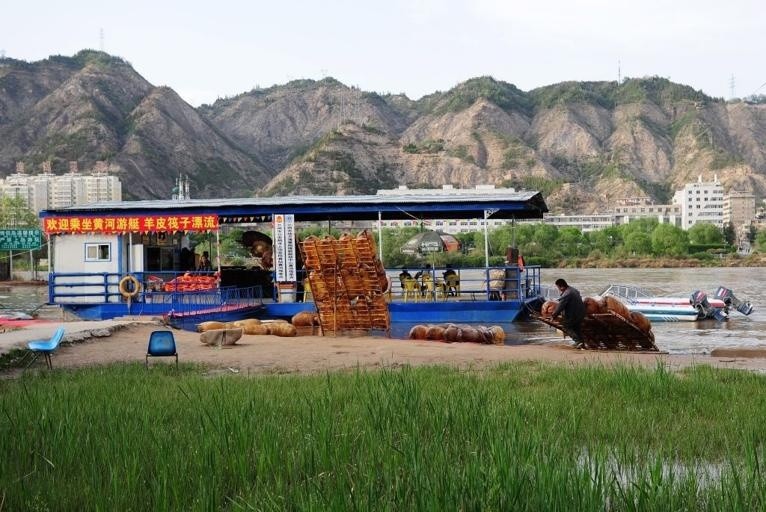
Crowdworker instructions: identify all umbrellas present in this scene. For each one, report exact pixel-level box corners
[399,230,464,289]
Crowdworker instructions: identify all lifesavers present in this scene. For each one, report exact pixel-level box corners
[119,276,140,298]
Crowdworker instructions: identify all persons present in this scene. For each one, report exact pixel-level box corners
[552,277,587,350]
[400,263,460,297]
[194,254,213,276]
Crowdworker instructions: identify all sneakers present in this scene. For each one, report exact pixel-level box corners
[576,341,583,349]
[580,347,586,350]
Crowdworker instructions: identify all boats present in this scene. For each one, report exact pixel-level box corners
[0,309,64,327]
[36,175,553,328]
[581,281,755,323]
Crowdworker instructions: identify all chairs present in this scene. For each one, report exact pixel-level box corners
[400,275,459,302]
[303,278,312,302]
[15,327,63,372]
[145,331,178,369]
